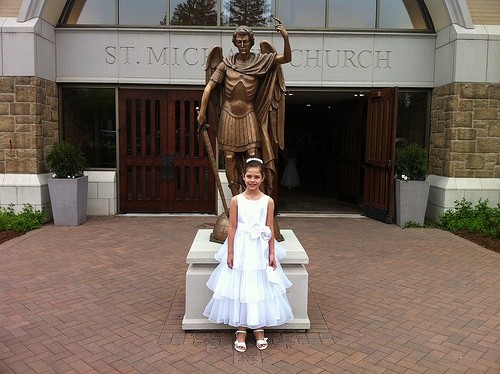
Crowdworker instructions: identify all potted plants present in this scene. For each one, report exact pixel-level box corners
[394,142,432,227]
[45,142,88,226]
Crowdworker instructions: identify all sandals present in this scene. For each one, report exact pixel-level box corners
[253,330,268,350]
[234,331,247,352]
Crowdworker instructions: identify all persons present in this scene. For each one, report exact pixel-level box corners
[201,156,296,353]
[196,15,292,242]
